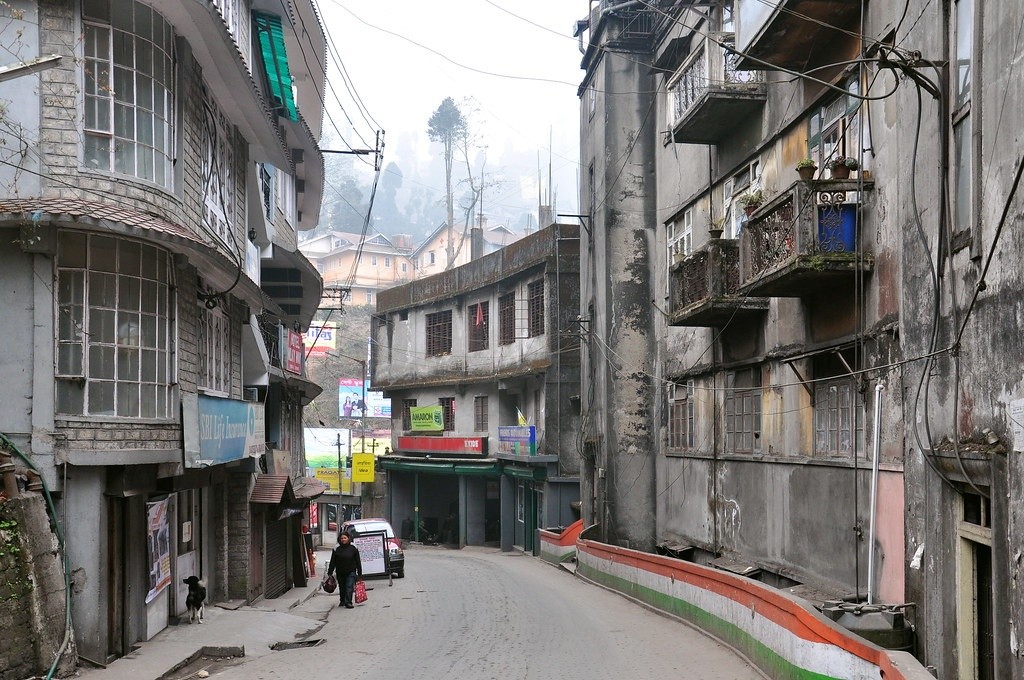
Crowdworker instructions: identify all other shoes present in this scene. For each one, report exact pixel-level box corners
[339,601,345,606]
[345,602,354,608]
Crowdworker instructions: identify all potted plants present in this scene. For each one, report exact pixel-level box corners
[795,159,818,178]
[744,192,762,216]
[671,243,690,262]
[825,155,857,179]
[703,210,726,238]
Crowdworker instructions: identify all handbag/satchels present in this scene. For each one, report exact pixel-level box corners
[355,579,368,604]
[323,575,337,593]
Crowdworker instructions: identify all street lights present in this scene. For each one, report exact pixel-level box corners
[324,350,365,451]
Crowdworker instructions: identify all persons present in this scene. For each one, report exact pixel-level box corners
[343,392,369,417]
[327,531,363,608]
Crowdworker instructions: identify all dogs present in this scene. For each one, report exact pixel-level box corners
[183,575,206,624]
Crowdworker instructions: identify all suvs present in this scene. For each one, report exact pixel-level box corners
[341,517,404,579]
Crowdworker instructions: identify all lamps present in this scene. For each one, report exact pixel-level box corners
[248,227,257,243]
[292,320,299,332]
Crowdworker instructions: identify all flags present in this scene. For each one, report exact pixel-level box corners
[476,303,483,329]
[518,411,526,426]
